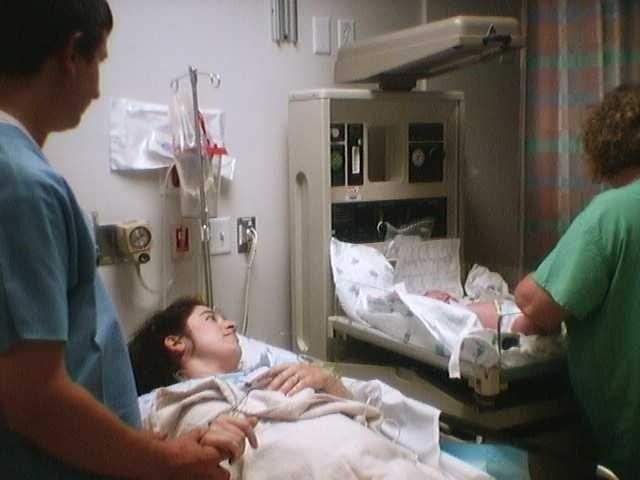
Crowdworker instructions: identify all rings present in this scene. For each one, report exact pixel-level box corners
[292,373,301,380]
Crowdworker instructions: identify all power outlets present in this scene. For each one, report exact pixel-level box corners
[237,216,256,254]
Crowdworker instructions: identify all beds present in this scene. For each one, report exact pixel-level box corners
[327,297,568,396]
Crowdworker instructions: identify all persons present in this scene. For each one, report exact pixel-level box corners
[513,83,640,480]
[422,288,562,337]
[0,0,231,479]
[129,294,355,465]
